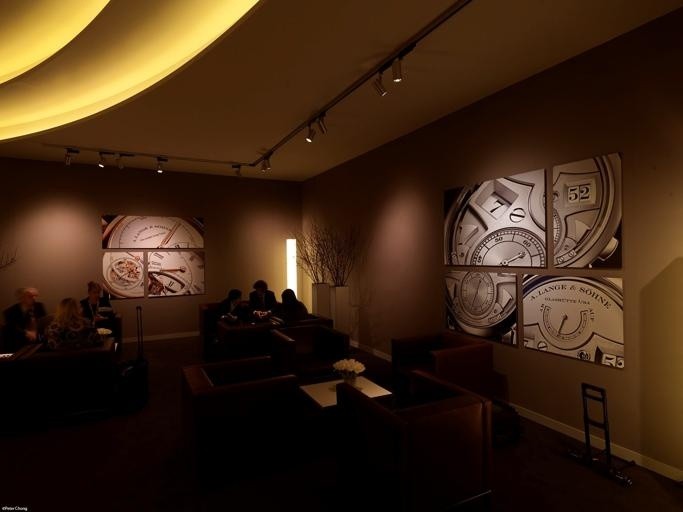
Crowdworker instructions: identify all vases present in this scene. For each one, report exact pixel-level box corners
[332,287,350,332]
[312,282,330,316]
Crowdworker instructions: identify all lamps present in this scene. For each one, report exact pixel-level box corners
[254,0,471,172]
[64,146,253,174]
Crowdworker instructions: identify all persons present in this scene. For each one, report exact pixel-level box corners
[277,290,309,323]
[2,287,46,353]
[215,290,243,322]
[244,280,278,320]
[46,298,104,351]
[80,281,116,335]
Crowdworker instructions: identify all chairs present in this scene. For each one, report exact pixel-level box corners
[0,312,123,436]
